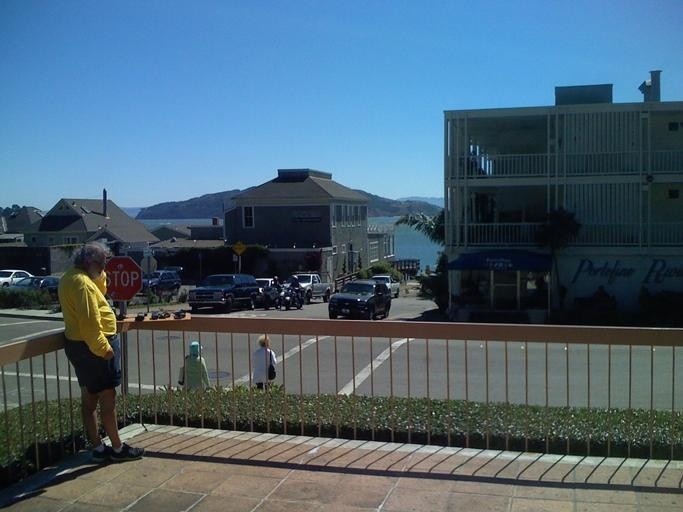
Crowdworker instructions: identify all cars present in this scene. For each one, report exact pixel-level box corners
[3,276,60,301]
[254,278,284,308]
[0,269,44,288]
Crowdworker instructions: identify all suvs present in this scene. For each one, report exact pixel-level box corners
[328,279,391,320]
[187,273,258,314]
[139,266,182,295]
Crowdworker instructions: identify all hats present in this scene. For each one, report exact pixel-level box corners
[189,341,203,354]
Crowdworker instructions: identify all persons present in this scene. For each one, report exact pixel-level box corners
[290,278,303,310]
[271,276,281,294]
[251,334,278,390]
[183,341,210,387]
[58,242,145,462]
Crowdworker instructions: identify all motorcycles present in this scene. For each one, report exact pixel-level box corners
[283,285,302,311]
[261,279,283,310]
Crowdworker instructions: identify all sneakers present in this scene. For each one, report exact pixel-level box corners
[109,442,144,461]
[90,443,112,461]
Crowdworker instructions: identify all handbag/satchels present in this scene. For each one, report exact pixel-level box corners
[177,355,188,385]
[267,350,276,380]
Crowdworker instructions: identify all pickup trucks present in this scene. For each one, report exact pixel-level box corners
[298,274,330,304]
[372,276,400,298]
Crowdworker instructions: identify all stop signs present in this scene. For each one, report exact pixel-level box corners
[103,256,142,302]
